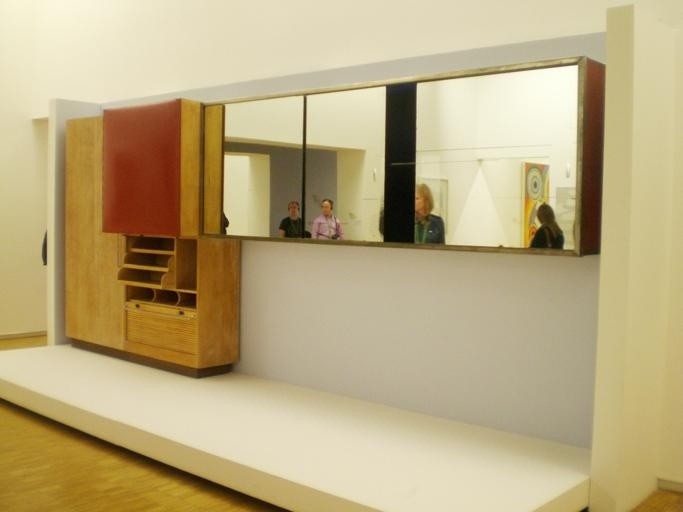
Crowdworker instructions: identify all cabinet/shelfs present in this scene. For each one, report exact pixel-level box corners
[102,98,223,238]
[125,236,241,378]
[63,115,124,361]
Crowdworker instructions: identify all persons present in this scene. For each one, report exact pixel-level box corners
[312,198,344,241]
[278,200,305,238]
[415,183,445,244]
[527,204,564,250]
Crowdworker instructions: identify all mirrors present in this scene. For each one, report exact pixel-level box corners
[302,80,413,250]
[220,90,303,243]
[412,55,605,258]
